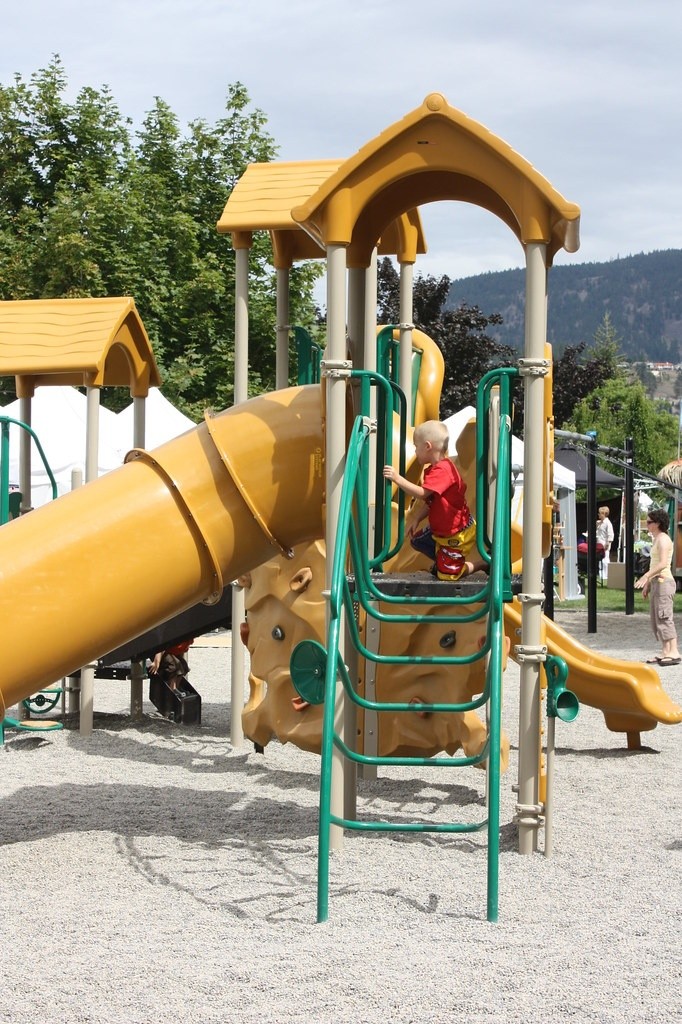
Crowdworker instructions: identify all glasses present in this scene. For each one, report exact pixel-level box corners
[647,520,657,525]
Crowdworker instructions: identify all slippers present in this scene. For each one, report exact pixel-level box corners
[646,656,682,667]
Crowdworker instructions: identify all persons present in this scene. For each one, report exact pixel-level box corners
[633,509,681,666]
[148,638,194,718]
[383,420,492,581]
[596,506,614,579]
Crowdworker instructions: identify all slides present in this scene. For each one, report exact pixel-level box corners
[0,383,327,721]
[504,596,682,732]
